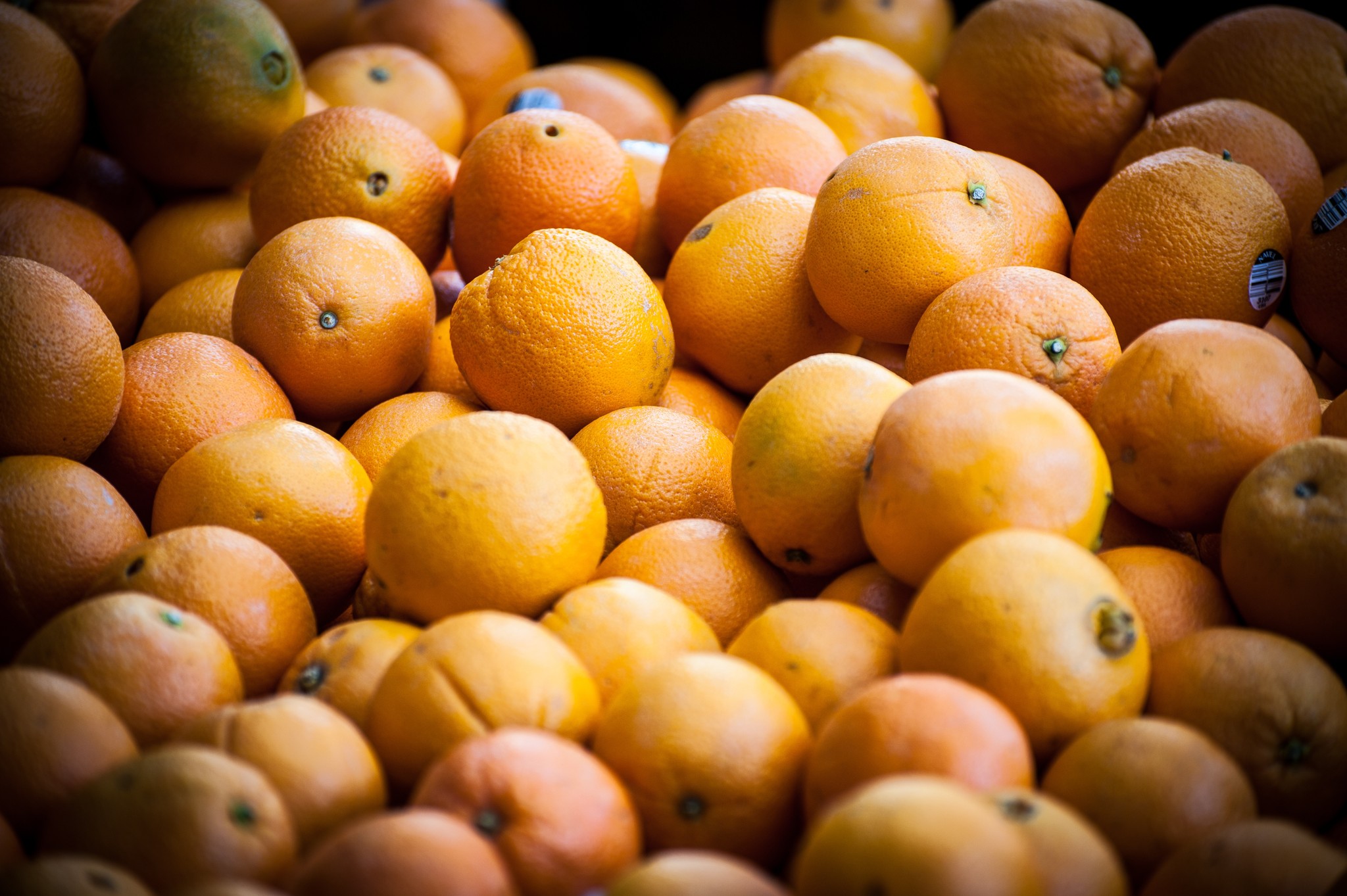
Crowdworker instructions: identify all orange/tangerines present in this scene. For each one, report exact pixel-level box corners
[3,0,1343,896]
